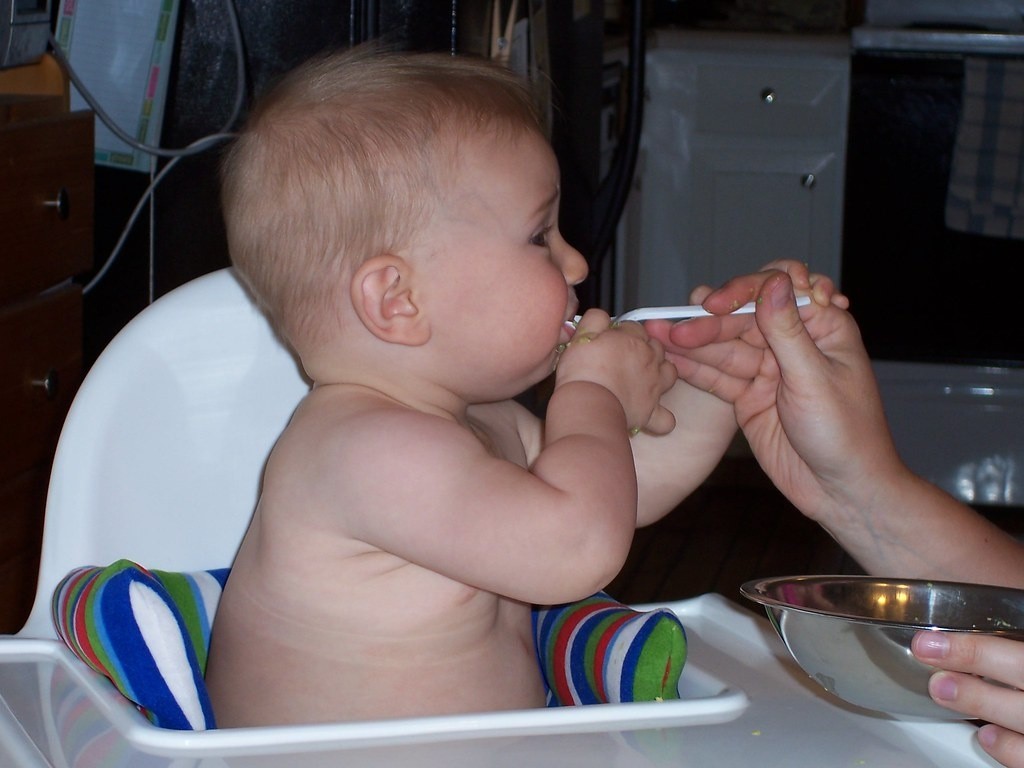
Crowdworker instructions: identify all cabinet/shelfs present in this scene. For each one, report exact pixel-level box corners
[0,92,97,635]
[597,24,854,315]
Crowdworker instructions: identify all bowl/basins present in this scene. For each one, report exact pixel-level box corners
[739,575,1024,719]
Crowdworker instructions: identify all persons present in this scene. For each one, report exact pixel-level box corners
[203,38,849,729]
[644,259,1024,768]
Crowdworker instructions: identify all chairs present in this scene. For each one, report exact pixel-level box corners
[0,258,1003,768]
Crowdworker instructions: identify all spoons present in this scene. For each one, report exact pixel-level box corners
[565,297,811,330]
